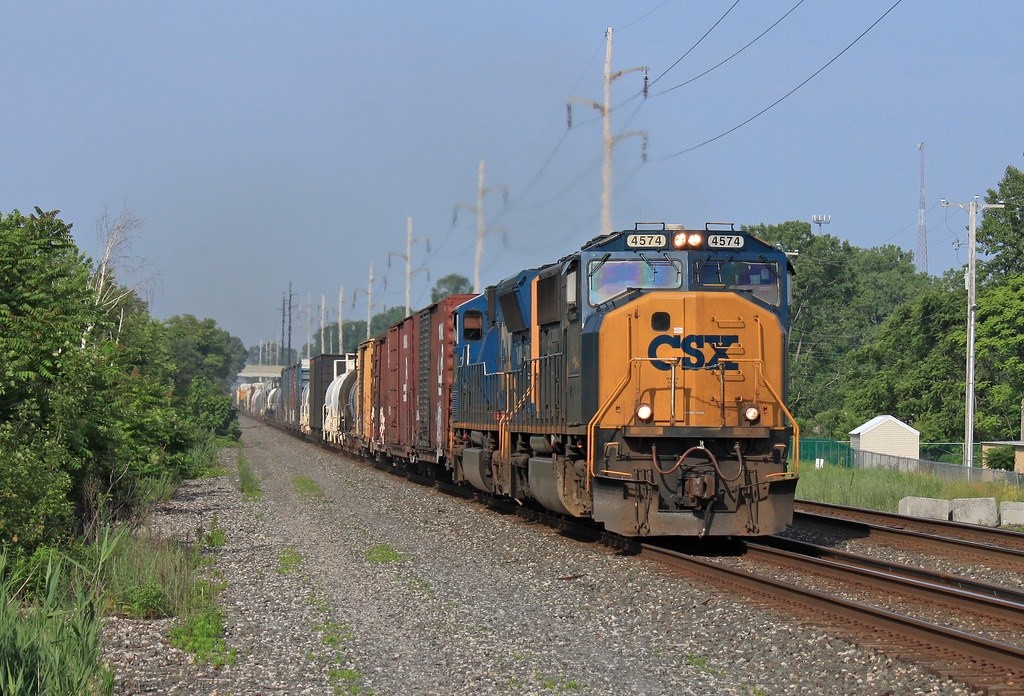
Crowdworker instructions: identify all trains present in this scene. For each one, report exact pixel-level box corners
[228,221,800,537]
